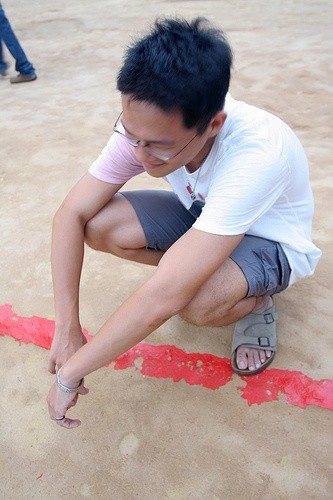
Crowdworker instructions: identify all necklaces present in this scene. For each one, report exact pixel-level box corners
[182,165,200,200]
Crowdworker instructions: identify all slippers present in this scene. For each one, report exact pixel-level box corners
[230,303,278,375]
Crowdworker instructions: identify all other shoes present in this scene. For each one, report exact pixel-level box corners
[9,73,37,84]
[0,70,10,79]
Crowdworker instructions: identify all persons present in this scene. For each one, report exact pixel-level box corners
[47,16,323,429]
[0,0,36,84]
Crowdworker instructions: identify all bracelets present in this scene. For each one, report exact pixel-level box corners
[56,367,84,392]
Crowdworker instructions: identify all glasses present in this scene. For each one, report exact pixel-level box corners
[112,110,208,162]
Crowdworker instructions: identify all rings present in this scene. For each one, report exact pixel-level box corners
[54,414,66,422]
